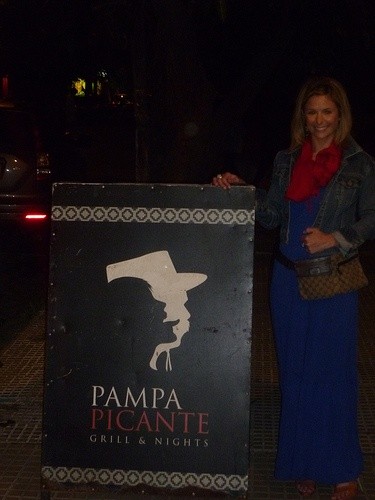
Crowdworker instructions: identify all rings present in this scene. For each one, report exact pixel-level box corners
[216,174,222,179]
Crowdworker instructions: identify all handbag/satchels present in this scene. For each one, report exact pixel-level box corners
[291,252,370,299]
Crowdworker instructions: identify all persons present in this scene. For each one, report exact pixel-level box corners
[211,75,375,500]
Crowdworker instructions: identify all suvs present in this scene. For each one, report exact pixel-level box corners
[0,97,94,259]
